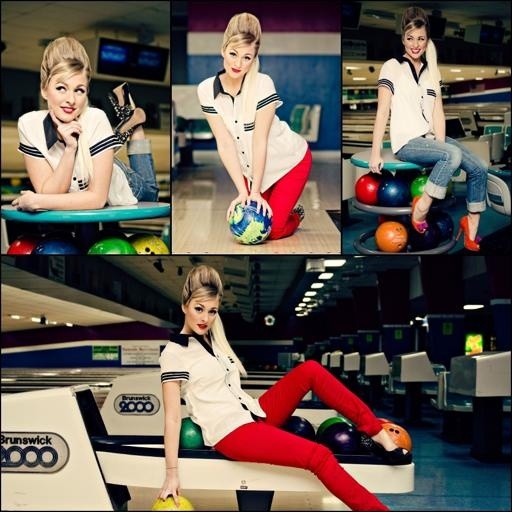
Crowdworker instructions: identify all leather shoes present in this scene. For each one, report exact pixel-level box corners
[293,205,305,223]
[361,434,409,460]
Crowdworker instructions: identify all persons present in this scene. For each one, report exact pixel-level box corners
[9,35,159,214]
[159,264,412,512]
[367,7,488,253]
[194,11,313,241]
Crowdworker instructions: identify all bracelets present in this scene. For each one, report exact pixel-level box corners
[164,465,179,472]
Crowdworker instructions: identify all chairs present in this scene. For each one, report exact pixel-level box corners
[171,84,214,168]
[477,125,511,168]
[285,103,321,144]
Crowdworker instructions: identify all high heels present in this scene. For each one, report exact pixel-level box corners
[455,216,480,252]
[410,196,428,234]
[108,82,147,143]
